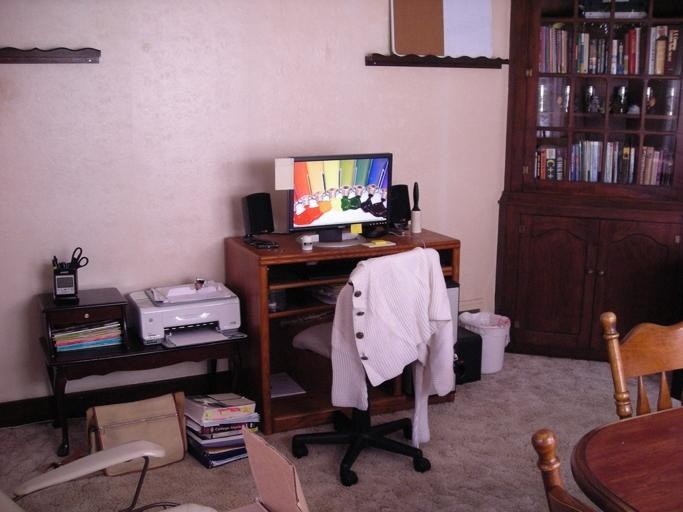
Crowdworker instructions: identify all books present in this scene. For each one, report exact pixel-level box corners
[538,24,681,75]
[184,391,261,468]
[51,319,122,352]
[534,139,673,186]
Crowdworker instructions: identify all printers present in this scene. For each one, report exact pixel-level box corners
[127,278,248,349]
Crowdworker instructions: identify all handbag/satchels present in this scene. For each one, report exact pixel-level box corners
[87,392,188,475]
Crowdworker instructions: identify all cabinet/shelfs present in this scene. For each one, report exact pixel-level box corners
[222,229,461,436]
[494,0,683,363]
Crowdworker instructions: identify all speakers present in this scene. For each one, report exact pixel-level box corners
[391,184,411,234]
[241,190,279,249]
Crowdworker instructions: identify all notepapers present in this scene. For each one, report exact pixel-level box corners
[373,240,385,244]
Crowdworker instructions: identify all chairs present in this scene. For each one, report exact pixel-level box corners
[598,311,683,419]
[527,428,602,512]
[0,440,222,511]
[290,247,442,487]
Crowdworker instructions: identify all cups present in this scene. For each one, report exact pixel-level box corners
[301,235,313,250]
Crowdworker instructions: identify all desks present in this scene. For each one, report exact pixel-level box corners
[569,405,682,512]
[37,331,250,458]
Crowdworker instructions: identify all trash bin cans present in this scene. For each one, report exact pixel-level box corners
[458,312,511,374]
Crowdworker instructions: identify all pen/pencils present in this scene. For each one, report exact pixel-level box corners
[52,255,67,274]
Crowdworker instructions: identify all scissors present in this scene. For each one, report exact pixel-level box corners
[69,247,88,274]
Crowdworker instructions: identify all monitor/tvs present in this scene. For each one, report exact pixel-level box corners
[289,154,394,248]
